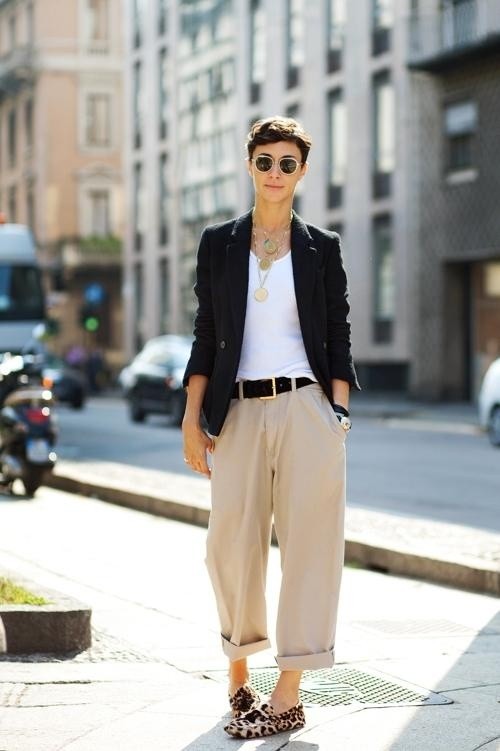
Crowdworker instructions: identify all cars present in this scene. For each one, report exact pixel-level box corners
[120,335,195,425]
[476,357,499,449]
[38,359,88,408]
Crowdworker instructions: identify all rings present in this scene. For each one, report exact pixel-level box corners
[183,458,190,463]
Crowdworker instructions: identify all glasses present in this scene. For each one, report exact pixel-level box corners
[250,155,303,175]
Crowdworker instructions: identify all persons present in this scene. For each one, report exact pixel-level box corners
[179,118,369,741]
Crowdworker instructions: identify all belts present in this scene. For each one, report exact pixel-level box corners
[232,376,316,400]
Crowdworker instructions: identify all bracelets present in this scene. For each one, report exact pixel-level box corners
[333,402,352,435]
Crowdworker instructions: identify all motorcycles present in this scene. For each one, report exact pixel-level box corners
[0,321,59,499]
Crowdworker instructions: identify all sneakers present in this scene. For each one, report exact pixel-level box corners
[227,685,260,718]
[223,701,305,739]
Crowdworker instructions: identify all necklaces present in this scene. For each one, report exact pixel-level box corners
[253,208,292,306]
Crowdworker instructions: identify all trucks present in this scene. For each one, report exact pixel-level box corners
[1,216,49,370]
